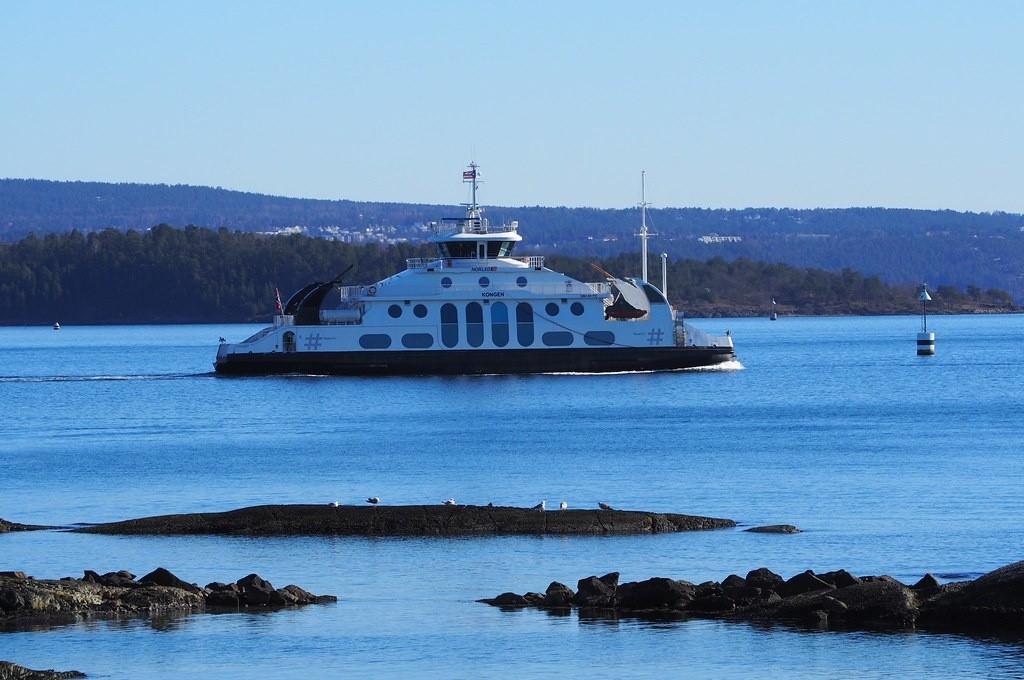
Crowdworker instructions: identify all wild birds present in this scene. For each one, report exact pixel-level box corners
[328,501,340,508]
[365,497,379,504]
[531,501,545,510]
[442,498,456,505]
[560,501,568,511]
[597,502,613,510]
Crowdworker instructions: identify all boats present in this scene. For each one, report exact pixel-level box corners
[211,160,737,377]
[605,305,647,318]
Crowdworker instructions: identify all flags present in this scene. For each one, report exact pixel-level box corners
[275,290,281,309]
